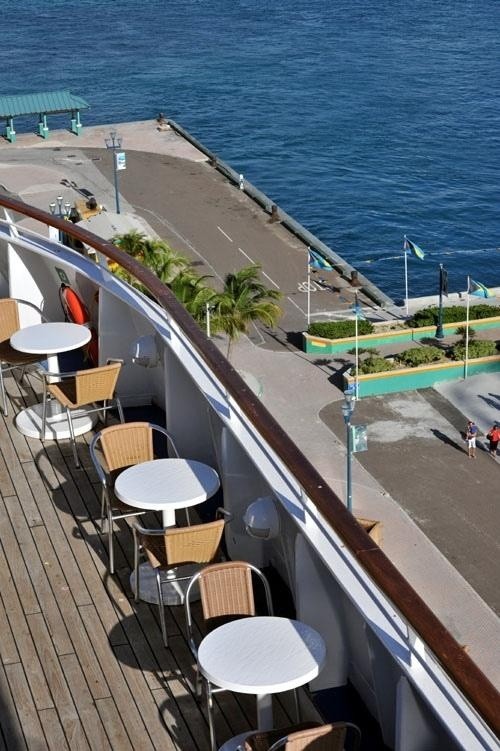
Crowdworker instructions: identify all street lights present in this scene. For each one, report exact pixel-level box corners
[49,195,71,244]
[104,130,123,214]
[339,389,356,512]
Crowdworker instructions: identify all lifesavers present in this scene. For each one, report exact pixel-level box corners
[61,287,99,364]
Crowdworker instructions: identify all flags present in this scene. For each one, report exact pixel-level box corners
[466,278,490,299]
[306,249,336,273]
[440,270,449,298]
[353,295,368,321]
[402,237,427,262]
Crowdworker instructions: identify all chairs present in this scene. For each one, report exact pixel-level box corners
[184,562,362,751]
[91,423,235,647]
[0,298,129,469]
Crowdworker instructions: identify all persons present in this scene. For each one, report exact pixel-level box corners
[466,422,477,460]
[488,425,500,455]
[463,424,478,441]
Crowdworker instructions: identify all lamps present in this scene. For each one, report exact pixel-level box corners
[132,331,165,371]
